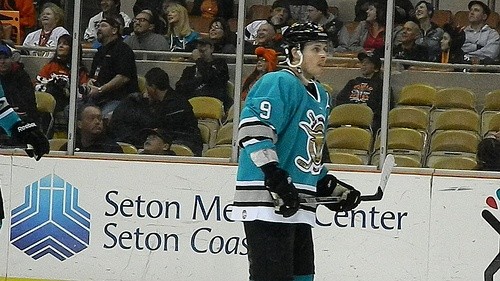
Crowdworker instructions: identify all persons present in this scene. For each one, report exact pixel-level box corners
[0,84,50,231]
[0,0,500,157]
[231,21,362,281]
[333,51,395,143]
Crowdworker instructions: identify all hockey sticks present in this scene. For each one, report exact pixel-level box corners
[0,144,34,150]
[274,154,395,207]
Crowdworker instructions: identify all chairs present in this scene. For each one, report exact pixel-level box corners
[35,5,500,169]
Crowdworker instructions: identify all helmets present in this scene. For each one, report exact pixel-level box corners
[283,22,328,48]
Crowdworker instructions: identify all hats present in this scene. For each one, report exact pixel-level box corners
[309,0,328,15]
[0,44,12,57]
[198,37,214,46]
[100,17,123,39]
[468,1,491,17]
[358,52,381,69]
[272,0,291,12]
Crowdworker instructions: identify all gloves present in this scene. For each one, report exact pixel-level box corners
[317,174,361,212]
[11,120,51,158]
[264,168,300,217]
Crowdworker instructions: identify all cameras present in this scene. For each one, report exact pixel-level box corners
[79,85,91,94]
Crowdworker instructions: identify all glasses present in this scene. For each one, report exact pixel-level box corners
[256,58,267,63]
[132,17,152,24]
